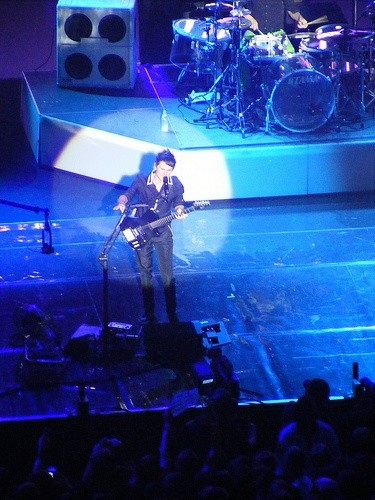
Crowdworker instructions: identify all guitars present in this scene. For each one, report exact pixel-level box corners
[120,200,211,250]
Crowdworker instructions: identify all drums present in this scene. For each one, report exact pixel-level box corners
[262,53,336,134]
[170,18,231,74]
[250,36,284,60]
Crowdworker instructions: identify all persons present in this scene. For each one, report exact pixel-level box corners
[113,148,185,325]
[0,379,375,500]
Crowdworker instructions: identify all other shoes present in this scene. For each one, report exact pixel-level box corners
[143,311,156,322]
[166,307,179,322]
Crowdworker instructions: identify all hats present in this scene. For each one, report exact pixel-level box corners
[303,378,331,398]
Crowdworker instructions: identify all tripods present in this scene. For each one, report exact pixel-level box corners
[174,0,265,138]
[331,37,375,129]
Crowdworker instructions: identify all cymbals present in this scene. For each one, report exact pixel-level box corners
[217,17,252,30]
[205,3,232,11]
[285,32,318,39]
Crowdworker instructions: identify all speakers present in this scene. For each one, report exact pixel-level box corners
[56,0,140,88]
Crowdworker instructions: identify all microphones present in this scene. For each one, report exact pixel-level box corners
[163,176,169,196]
